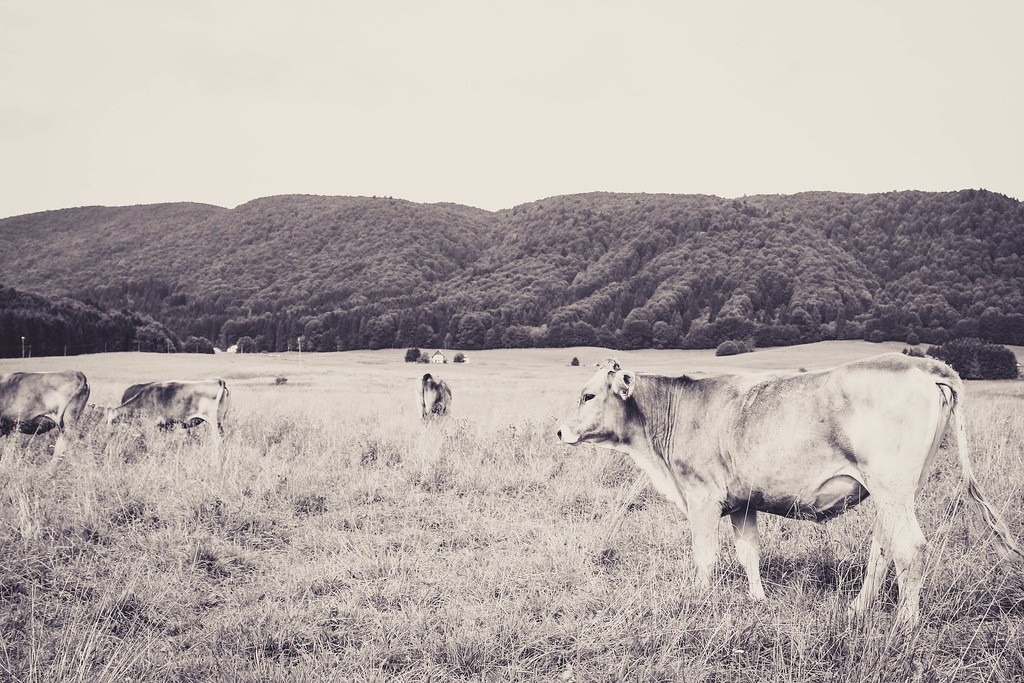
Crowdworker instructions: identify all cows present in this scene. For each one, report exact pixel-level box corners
[419,373,452,426]
[558,351,1024,636]
[88,379,230,452]
[0,371,91,464]
